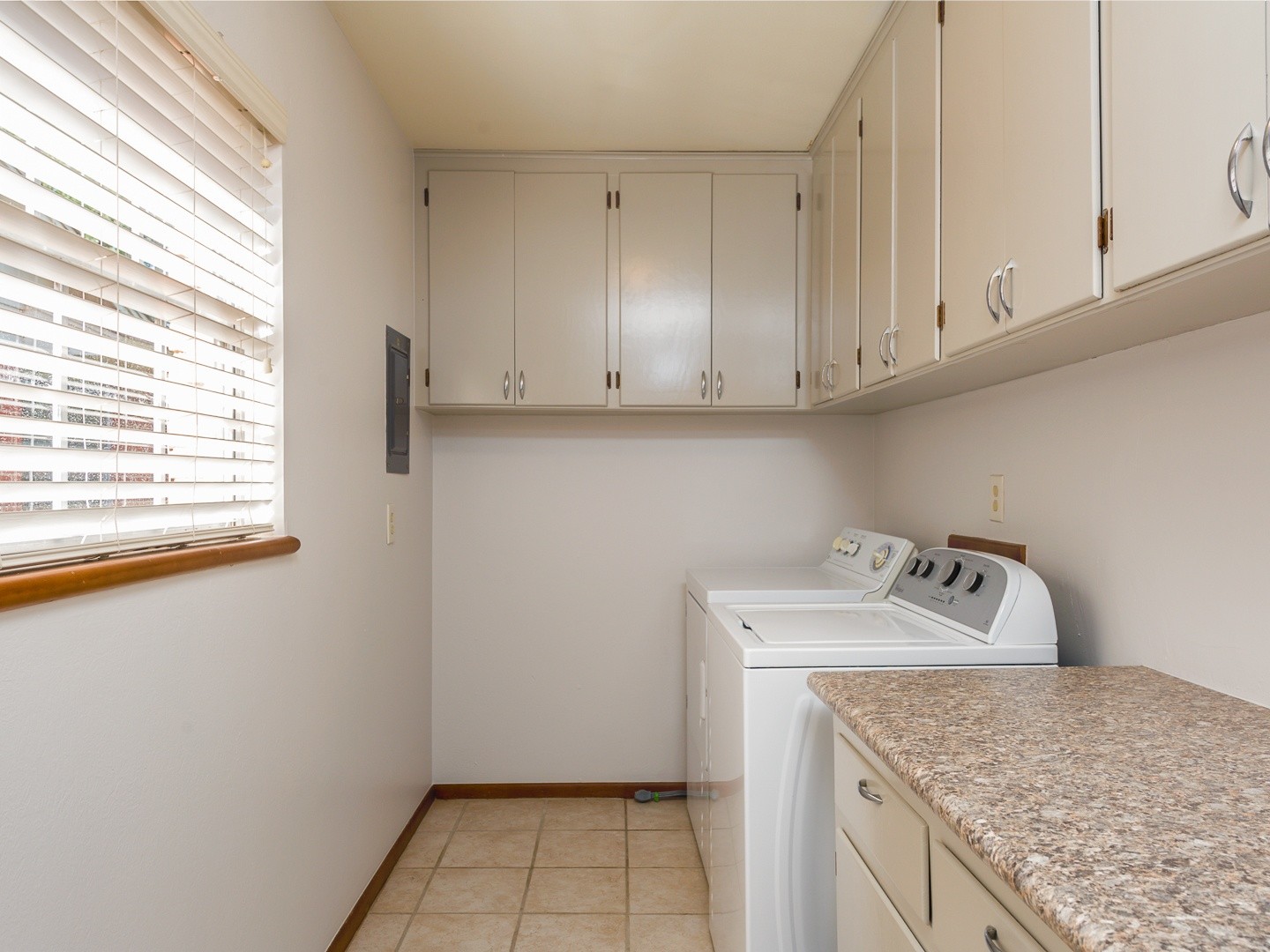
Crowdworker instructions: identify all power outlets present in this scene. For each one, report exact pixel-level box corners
[990,475,1004,521]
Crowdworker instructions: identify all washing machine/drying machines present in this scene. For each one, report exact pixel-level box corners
[704,545,1058,951]
[682,525,919,886]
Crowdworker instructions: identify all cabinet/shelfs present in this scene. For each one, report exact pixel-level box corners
[813,4,1244,412]
[835,718,1060,952]
[427,147,811,418]
